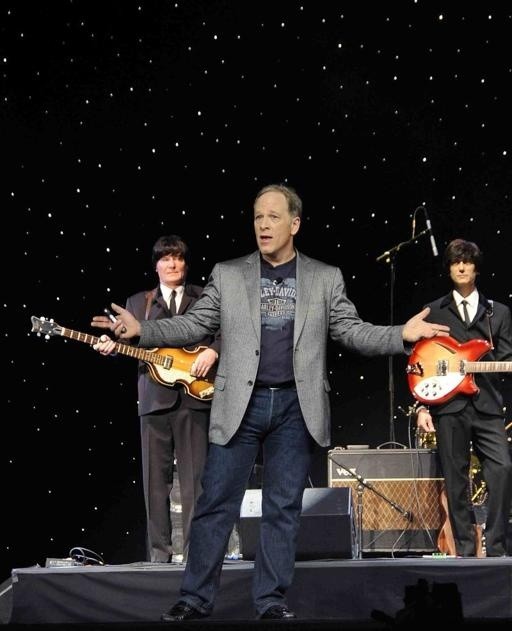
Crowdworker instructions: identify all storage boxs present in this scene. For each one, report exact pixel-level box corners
[327,447,443,554]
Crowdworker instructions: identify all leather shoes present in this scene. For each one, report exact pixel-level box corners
[260,605,298,619]
[160,600,208,622]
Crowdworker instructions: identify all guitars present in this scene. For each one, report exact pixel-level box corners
[407,337,512,406]
[31,315,220,400]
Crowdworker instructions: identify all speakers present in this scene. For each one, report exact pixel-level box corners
[238,485,358,562]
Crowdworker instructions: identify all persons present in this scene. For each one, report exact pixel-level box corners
[413,238,512,558]
[91,235,221,567]
[91,185,449,622]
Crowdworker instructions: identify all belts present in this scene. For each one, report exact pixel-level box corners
[254,379,295,391]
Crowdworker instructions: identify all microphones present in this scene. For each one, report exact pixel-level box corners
[421,203,442,258]
[104,307,127,334]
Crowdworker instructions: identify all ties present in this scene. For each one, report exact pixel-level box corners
[170,290,176,317]
[461,300,470,325]
